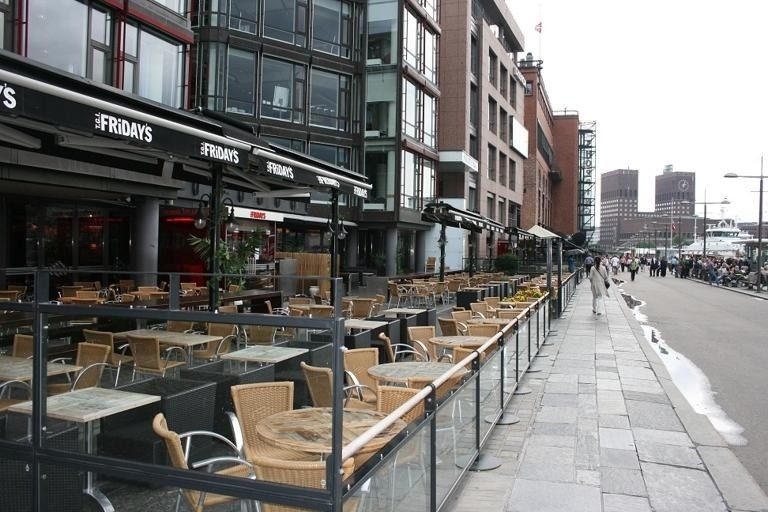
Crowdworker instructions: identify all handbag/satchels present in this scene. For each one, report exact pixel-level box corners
[604,279,610,289]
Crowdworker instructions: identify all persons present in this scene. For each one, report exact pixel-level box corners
[588,255,611,316]
[567,252,767,288]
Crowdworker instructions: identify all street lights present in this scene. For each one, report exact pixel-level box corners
[681,198,731,258]
[724,172,767,292]
[662,213,676,249]
[620,221,660,254]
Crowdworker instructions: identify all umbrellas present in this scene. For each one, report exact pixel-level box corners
[564,249,585,256]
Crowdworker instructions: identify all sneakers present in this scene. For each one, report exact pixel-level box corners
[593,310,601,315]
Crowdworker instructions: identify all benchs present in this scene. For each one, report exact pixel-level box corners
[741,272,759,290]
